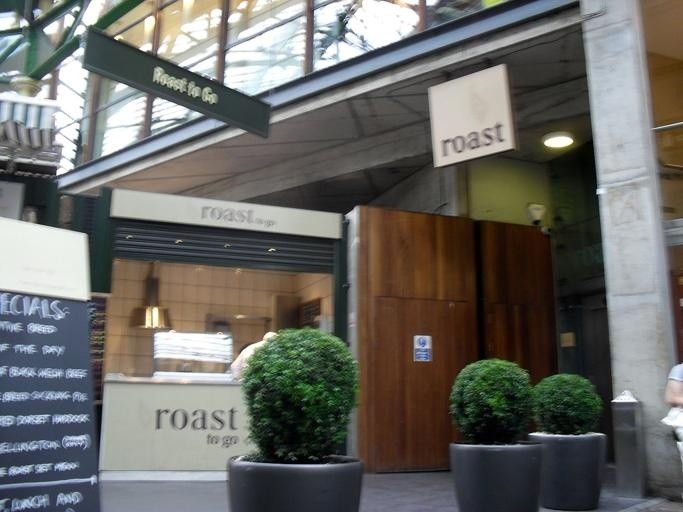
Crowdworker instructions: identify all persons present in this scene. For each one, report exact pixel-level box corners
[662,362,683,466]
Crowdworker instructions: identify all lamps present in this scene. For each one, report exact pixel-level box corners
[540,131,577,149]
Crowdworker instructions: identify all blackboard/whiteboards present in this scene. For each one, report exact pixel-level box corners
[0,289,99,512]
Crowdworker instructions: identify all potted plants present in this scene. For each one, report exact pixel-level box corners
[447,357,608,512]
[227,326,364,512]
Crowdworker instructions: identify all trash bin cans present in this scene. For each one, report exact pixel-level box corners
[610,390,646,498]
